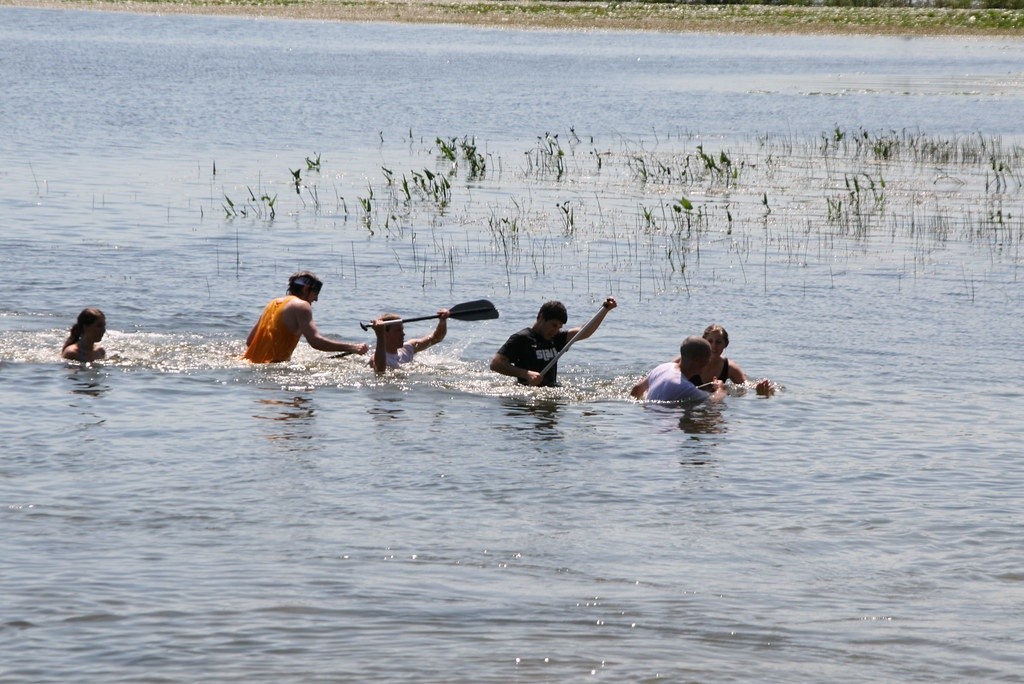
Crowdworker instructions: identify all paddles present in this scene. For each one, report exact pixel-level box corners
[361,299,500,334]
[528,300,613,390]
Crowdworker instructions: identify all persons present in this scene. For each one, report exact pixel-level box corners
[489,296,618,387]
[62,308,107,363]
[674,324,774,390]
[370,309,449,371]
[630,336,725,401]
[239,271,369,365]
